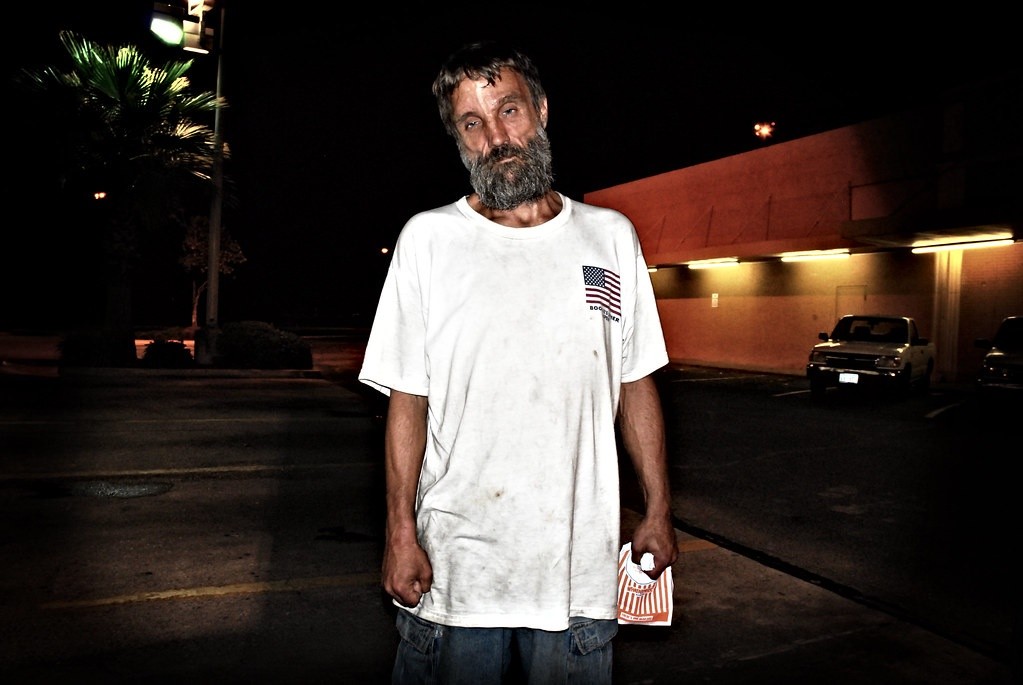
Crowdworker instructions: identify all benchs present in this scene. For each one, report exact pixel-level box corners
[780,253,852,262]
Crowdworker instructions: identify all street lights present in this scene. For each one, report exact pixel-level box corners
[147,0,226,365]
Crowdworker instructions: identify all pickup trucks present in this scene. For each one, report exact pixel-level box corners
[804,313,937,395]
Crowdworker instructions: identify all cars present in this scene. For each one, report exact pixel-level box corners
[971,316,1023,405]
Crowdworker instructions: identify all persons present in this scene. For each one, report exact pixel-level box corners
[358,40,680,685]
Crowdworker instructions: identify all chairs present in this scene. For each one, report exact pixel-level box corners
[854,326,903,341]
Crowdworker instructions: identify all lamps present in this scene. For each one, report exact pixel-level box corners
[648,268,658,273]
[910,239,1016,255]
[687,261,740,270]
